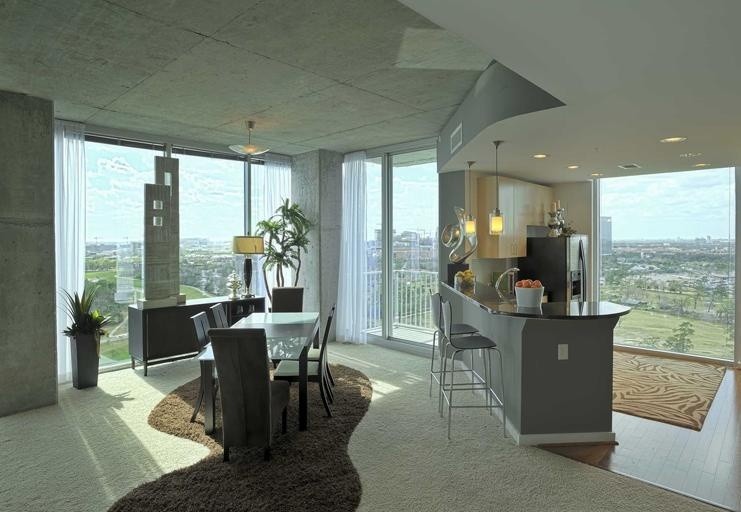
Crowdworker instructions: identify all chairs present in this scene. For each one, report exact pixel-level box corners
[439,298,510,441]
[428,288,492,412]
[188,287,337,461]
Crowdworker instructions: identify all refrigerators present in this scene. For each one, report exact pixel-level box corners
[518,234,589,302]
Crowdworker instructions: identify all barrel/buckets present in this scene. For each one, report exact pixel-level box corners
[515,286,544,310]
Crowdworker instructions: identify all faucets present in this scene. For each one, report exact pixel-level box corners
[492,266,522,301]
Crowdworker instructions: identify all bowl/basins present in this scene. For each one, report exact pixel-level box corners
[516,287,546,306]
[455,276,476,289]
[517,306,544,315]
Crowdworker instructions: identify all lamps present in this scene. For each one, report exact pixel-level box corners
[488,139,506,236]
[229,118,271,157]
[233,235,265,299]
[464,159,479,238]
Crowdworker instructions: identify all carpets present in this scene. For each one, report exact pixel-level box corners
[109,361,373,512]
[613,347,727,432]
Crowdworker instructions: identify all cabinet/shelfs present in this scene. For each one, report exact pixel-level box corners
[528,181,558,228]
[478,176,528,259]
[128,293,266,376]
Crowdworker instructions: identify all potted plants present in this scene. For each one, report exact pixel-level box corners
[56,282,116,389]
[246,195,320,313]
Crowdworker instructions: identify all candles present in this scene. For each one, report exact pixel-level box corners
[558,199,560,208]
[551,200,557,213]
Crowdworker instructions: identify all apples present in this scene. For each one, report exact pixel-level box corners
[455,270,474,278]
[515,279,541,288]
